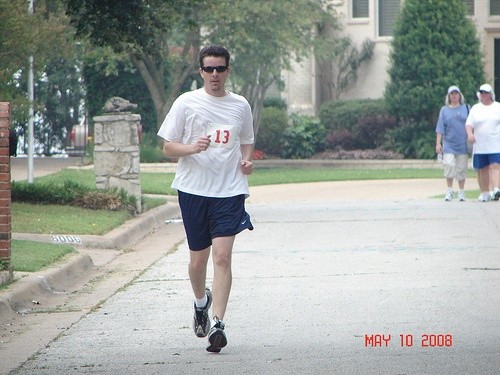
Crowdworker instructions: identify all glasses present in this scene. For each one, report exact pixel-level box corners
[201,65,229,72]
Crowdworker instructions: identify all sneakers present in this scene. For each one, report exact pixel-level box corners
[482,192,491,202]
[445,191,453,201]
[458,192,464,201]
[205,315,227,353]
[193,288,212,337]
[493,189,500,200]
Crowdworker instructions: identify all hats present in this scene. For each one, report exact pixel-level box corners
[479,84,491,93]
[448,85,459,94]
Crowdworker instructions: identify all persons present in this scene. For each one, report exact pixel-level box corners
[156,45,255,352]
[435,85,472,201]
[465,84,500,202]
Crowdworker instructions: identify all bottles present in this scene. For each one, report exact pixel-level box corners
[437,153,442,162]
